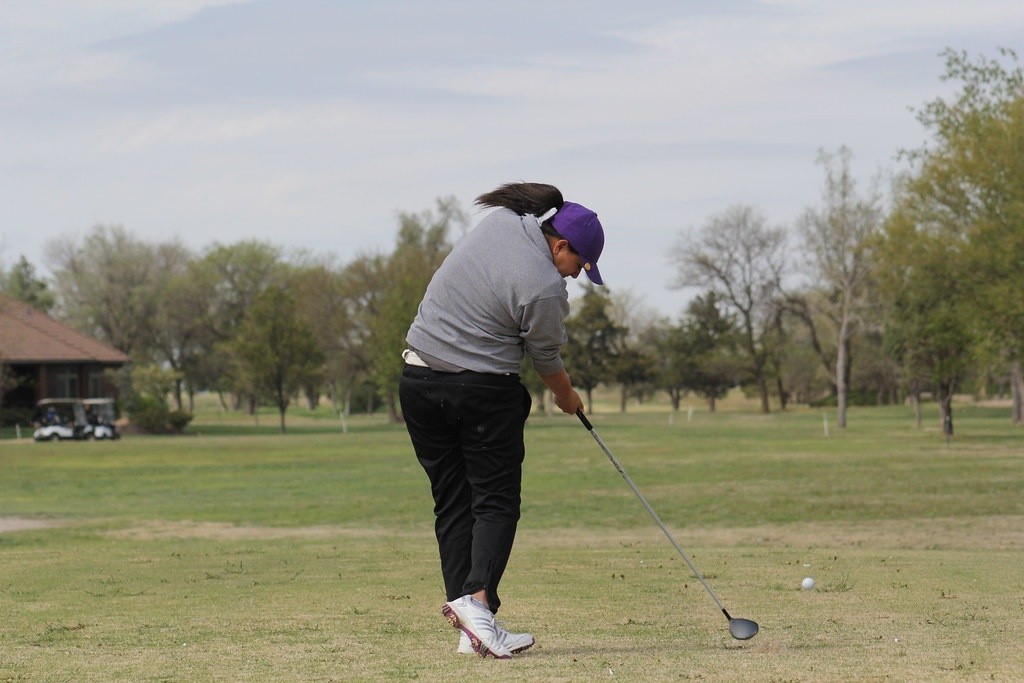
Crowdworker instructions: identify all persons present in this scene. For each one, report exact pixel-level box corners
[399,183,605,660]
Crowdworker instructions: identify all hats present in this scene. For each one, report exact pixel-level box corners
[552,201,604,285]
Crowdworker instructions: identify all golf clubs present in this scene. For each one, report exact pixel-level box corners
[573,407,760,641]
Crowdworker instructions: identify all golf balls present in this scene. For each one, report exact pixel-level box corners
[801,577,815,591]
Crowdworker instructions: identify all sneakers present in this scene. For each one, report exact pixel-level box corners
[457,623,534,656]
[442,594,513,659]
